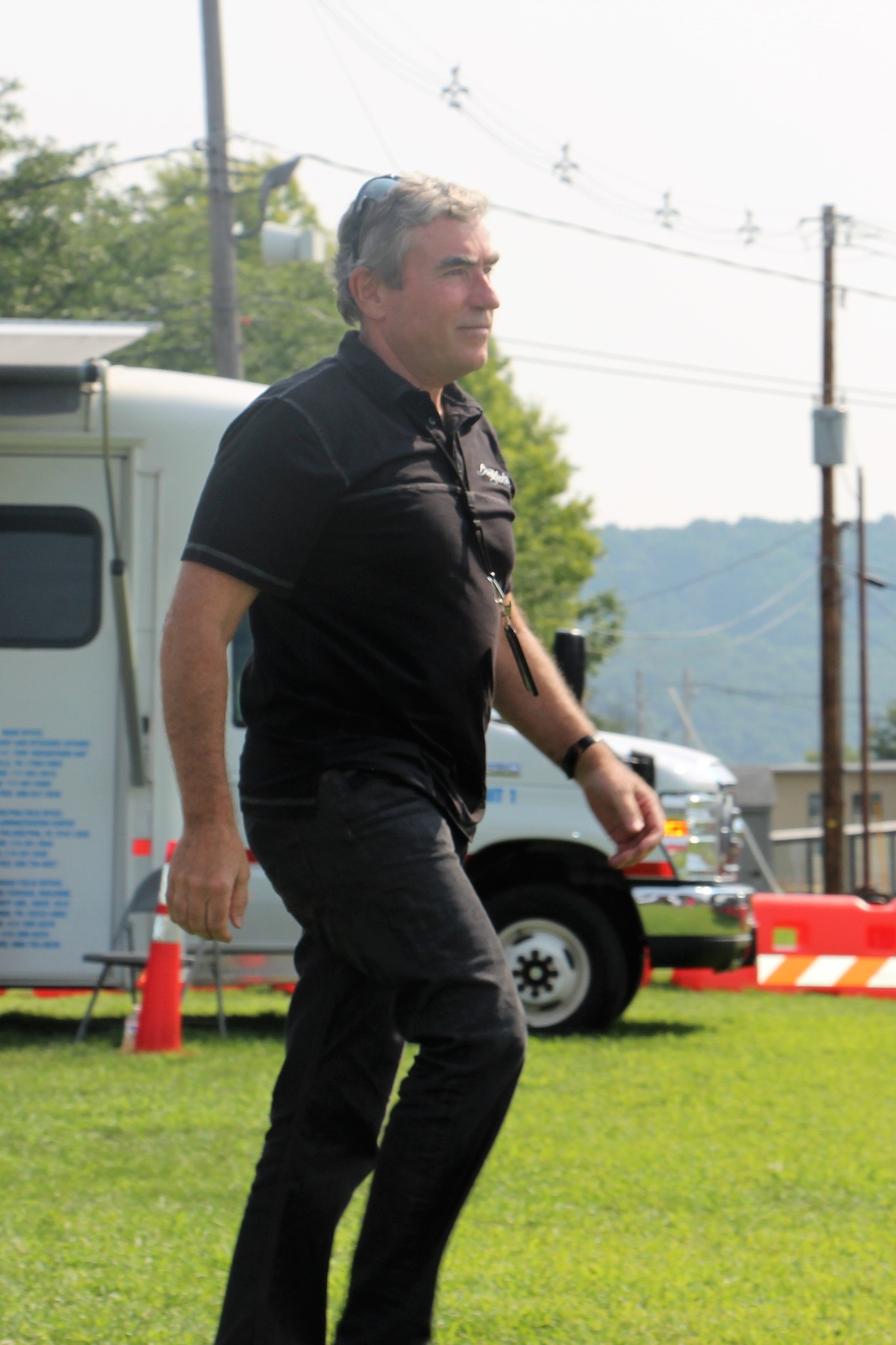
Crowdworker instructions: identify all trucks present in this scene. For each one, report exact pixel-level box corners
[1,315,758,1043]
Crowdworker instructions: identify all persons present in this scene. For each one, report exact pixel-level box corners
[160,174,664,1345]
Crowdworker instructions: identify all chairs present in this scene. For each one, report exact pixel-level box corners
[77,867,229,1051]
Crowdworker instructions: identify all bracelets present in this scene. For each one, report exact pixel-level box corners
[561,735,602,779]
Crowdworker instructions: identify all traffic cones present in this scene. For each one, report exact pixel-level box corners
[133,840,183,1051]
